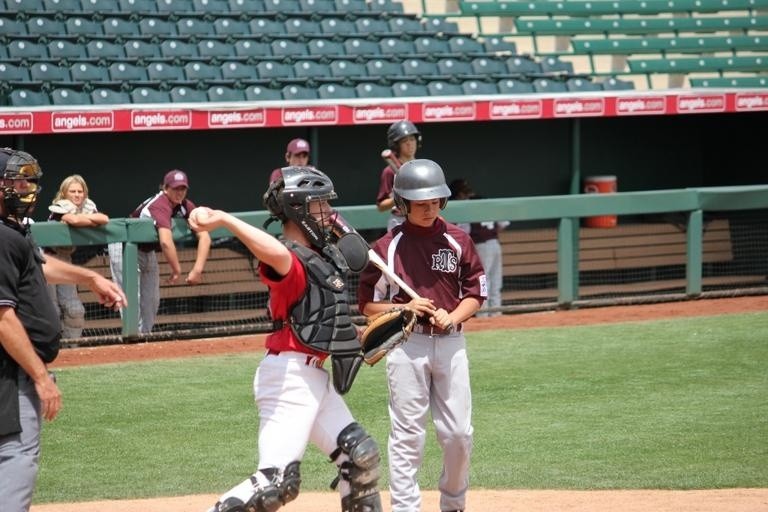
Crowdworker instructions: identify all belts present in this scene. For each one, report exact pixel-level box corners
[412,322,464,335]
[267,347,326,369]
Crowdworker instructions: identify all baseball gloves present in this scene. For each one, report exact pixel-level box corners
[358,305,416,366]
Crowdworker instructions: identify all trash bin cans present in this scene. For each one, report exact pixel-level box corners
[583,175,618,228]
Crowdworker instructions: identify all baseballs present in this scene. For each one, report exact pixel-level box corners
[189,206,210,225]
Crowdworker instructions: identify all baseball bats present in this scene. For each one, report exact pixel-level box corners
[381,148,401,174]
[328,212,455,336]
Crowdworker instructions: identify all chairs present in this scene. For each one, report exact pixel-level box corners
[0,0,768,111]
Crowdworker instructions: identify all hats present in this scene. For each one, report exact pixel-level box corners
[163,169,190,189]
[287,138,310,154]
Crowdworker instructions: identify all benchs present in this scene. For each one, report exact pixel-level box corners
[76,214,766,332]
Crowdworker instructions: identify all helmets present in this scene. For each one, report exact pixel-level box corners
[0,147,44,180]
[263,165,340,206]
[387,119,421,144]
[392,157,455,203]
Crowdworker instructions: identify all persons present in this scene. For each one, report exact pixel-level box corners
[187,166,416,512]
[107,169,212,334]
[0,148,128,512]
[270,138,311,186]
[45,175,108,347]
[358,160,488,512]
[375,120,422,233]
[449,178,509,318]
